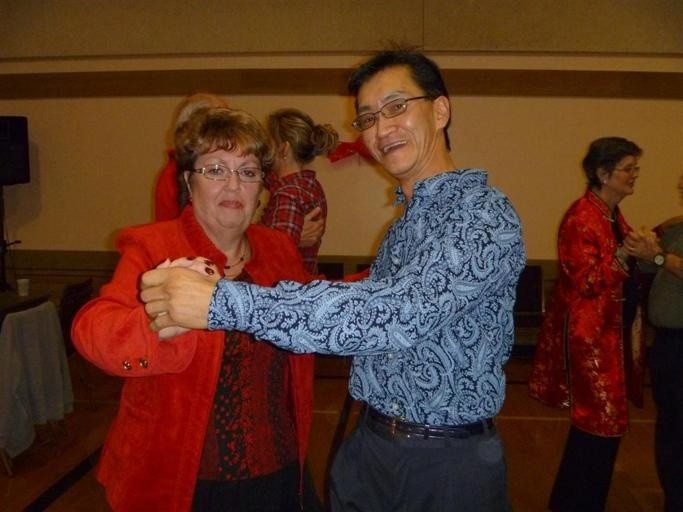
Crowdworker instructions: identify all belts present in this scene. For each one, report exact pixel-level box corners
[367,406,492,439]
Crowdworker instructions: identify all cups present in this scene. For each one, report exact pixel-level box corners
[16,276,32,298]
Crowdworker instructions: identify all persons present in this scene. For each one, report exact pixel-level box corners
[153,93,230,221]
[245,109,338,283]
[528,136,658,512]
[70,109,372,512]
[139,46,527,512]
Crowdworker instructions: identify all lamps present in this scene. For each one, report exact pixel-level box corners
[0,115,33,250]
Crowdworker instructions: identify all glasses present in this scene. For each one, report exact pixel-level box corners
[192,164,267,183]
[351,95,428,131]
[614,165,640,174]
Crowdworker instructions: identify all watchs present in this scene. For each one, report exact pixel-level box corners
[653,248,668,270]
[615,246,632,263]
[625,166,683,511]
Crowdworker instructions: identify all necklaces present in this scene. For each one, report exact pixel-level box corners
[225,237,250,272]
